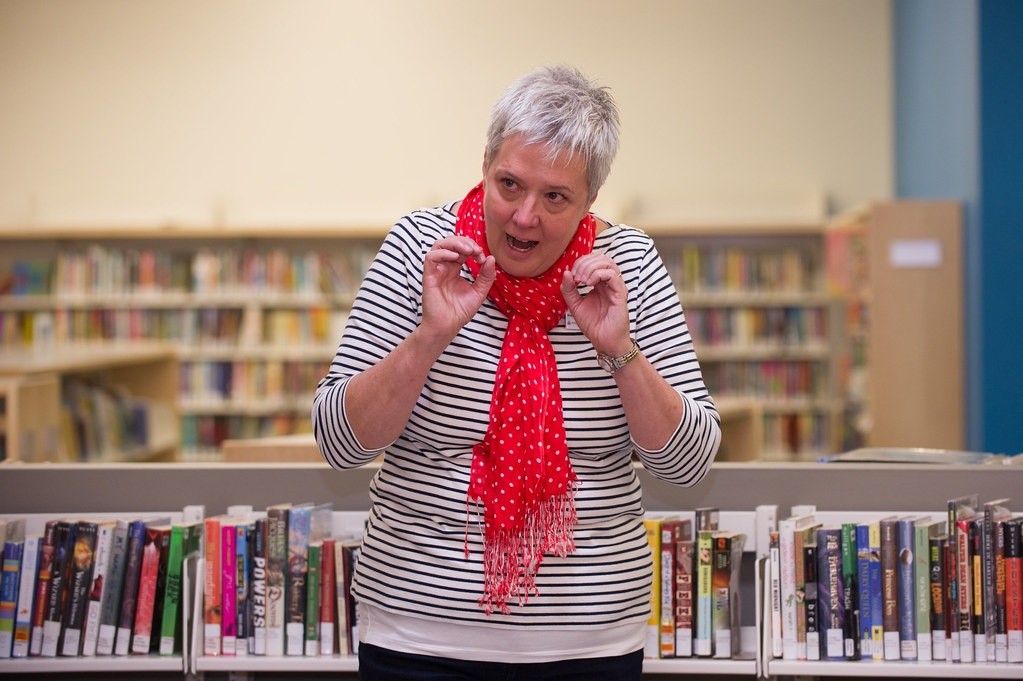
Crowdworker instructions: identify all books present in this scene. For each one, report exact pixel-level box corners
[728,412,829,456]
[644,494,1023,666]
[60,376,157,461]
[0,242,366,295]
[719,362,822,399]
[1,309,349,348]
[181,416,311,455]
[177,360,329,404]
[0,504,361,658]
[684,307,822,348]
[658,241,806,292]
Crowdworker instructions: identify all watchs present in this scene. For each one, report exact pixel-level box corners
[596,338,641,374]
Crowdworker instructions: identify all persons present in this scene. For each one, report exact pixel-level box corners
[311,59,721,681]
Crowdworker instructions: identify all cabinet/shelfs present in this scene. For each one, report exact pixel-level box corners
[0,198,1023,681]
